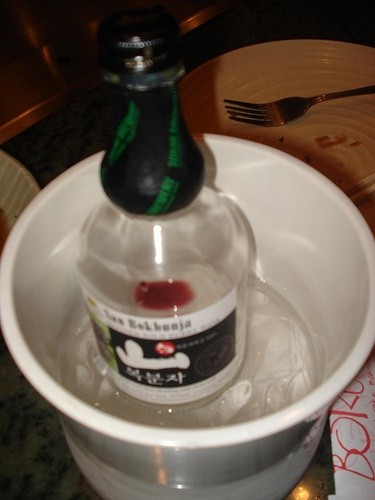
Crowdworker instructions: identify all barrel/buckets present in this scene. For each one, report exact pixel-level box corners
[0,131,374,500]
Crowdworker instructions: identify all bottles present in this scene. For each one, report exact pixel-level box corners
[74,20,257,414]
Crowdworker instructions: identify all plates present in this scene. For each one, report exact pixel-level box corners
[177,39,375,239]
[0,149,40,261]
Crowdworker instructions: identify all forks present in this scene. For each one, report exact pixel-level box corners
[222,85,375,126]
